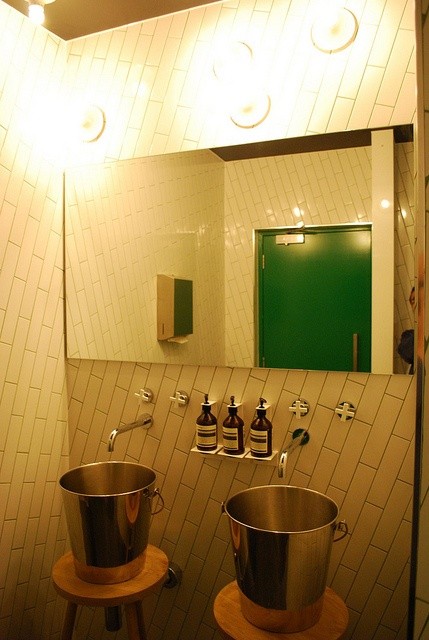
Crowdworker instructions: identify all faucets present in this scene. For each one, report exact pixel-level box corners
[276,432,312,479]
[107,412,153,452]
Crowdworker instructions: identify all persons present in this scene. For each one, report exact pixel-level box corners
[395,284,414,374]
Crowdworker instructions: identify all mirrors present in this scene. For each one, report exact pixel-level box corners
[64,127,413,377]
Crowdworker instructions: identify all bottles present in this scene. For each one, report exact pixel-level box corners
[221,396,247,458]
[194,393,218,456]
[249,396,272,462]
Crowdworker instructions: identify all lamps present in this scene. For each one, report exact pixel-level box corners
[27,0,48,29]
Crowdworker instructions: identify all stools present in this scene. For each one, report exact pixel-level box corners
[211,581,349,640]
[51,546,169,640]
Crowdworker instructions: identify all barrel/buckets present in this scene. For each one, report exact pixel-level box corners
[59,461,164,585]
[221,485,347,635]
